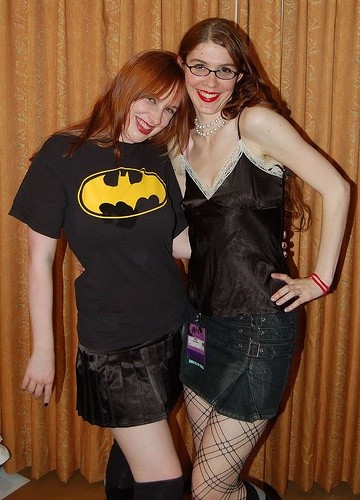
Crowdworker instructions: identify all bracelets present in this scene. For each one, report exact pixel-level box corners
[308,274,327,296]
[311,273,332,292]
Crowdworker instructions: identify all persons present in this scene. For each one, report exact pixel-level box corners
[7,49,288,500]
[164,16,351,500]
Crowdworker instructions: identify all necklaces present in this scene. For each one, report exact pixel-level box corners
[194,114,226,129]
[195,117,230,136]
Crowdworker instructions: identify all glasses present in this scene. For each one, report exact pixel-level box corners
[183,60,240,79]
